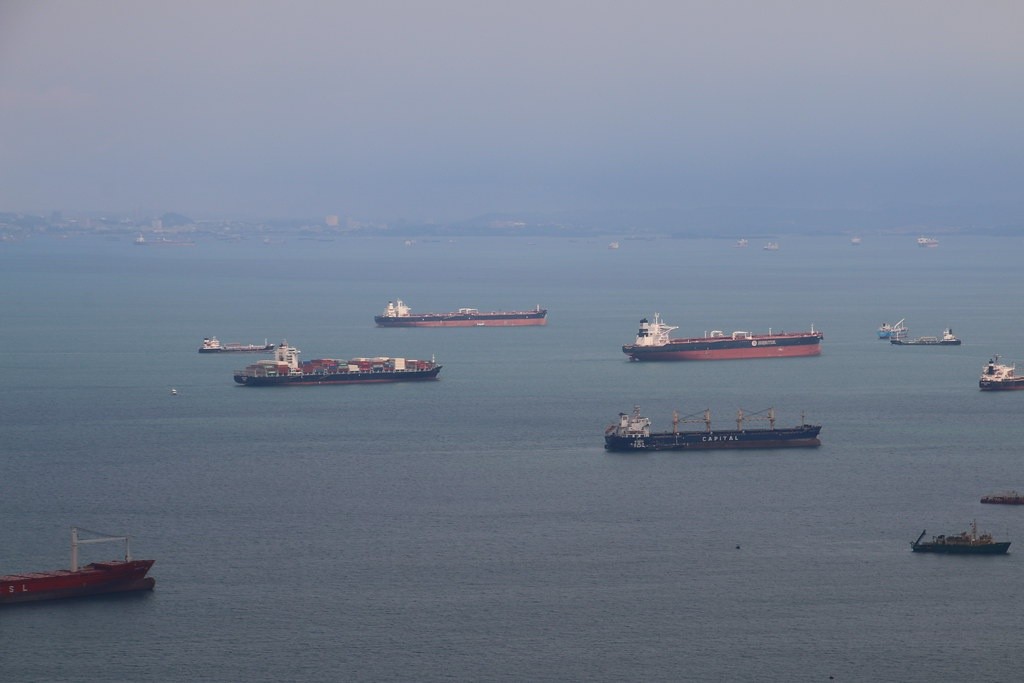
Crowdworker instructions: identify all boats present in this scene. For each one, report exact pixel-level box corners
[374,296,549,328]
[197,334,276,354]
[877,318,908,339]
[232,336,443,386]
[620,310,824,363]
[909,518,1012,554]
[0,528,156,605]
[889,328,961,346]
[978,354,1024,390]
[602,404,822,453]
[979,492,1024,505]
